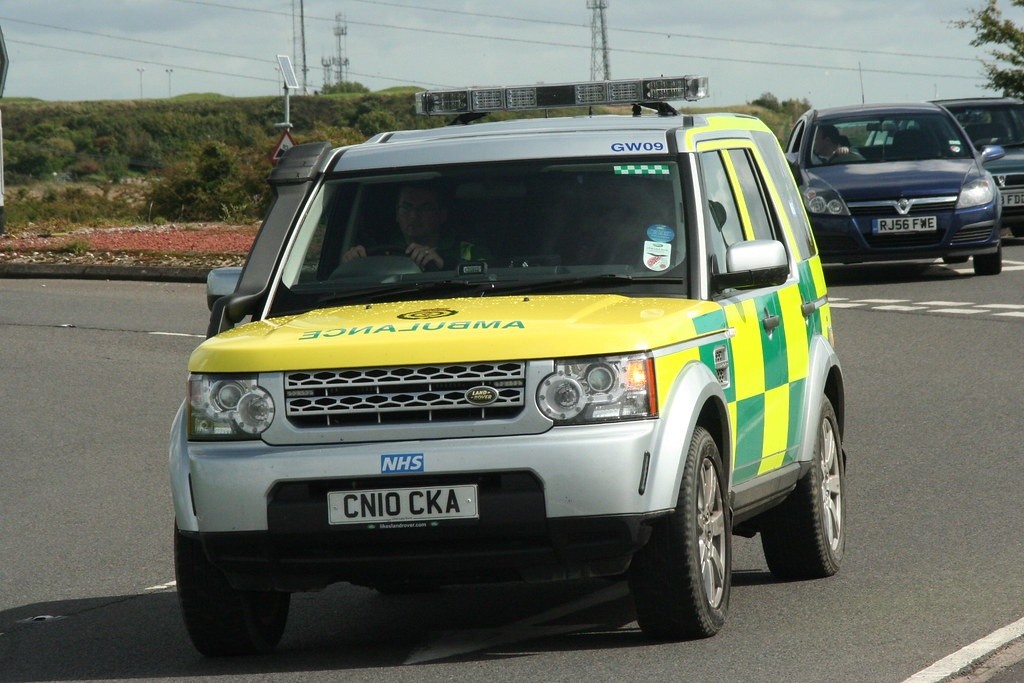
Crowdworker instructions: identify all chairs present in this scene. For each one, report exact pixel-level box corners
[893,129,936,157]
[965,123,1010,148]
[587,178,677,265]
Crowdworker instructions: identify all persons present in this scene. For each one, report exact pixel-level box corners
[812,125,849,164]
[340,185,488,269]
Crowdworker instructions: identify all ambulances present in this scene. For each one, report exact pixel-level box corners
[163,68,848,655]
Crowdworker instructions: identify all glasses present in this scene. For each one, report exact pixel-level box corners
[397,204,438,218]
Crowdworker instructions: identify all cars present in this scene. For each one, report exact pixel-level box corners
[861,97,1024,239]
[773,101,1007,280]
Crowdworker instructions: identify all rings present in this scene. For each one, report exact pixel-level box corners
[423,249,431,255]
